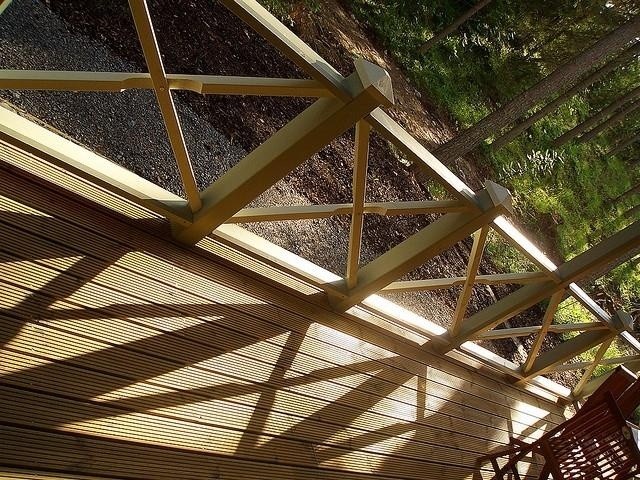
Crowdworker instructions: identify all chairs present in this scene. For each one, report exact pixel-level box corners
[477,391,640,480]
